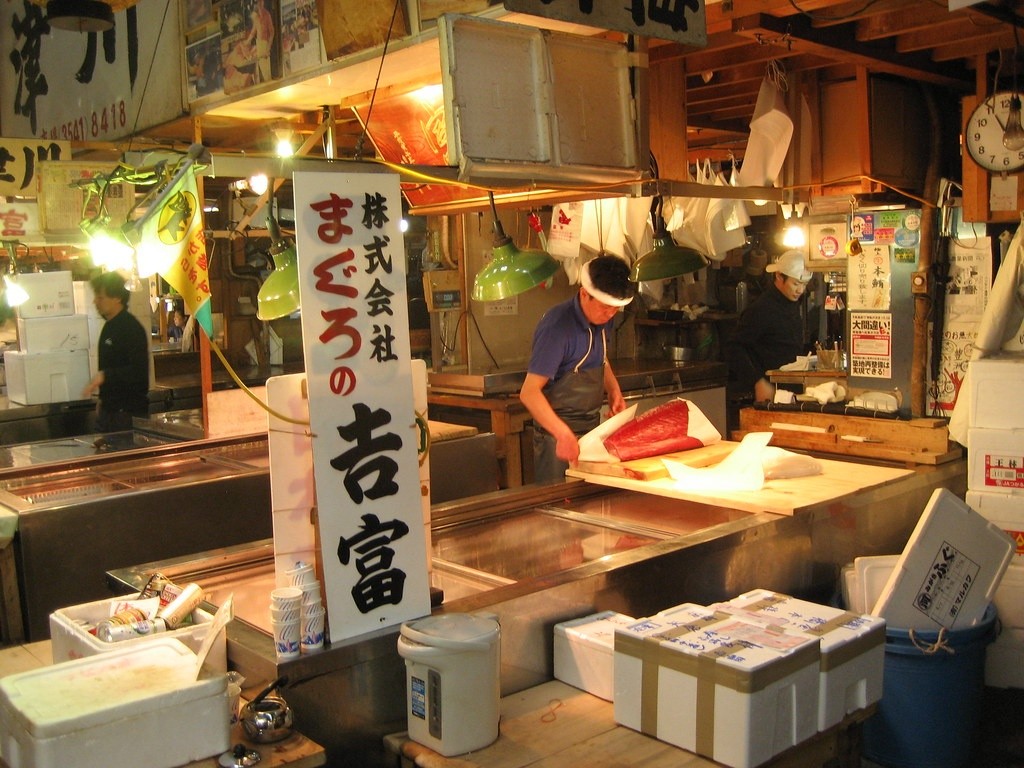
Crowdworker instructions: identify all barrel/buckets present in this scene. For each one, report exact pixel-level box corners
[830,591,998,768]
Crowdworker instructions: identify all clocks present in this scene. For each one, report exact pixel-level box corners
[965,91,1024,174]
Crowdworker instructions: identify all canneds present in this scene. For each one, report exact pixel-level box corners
[71,571,205,643]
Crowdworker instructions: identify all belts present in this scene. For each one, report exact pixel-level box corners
[111,406,134,414]
[535,426,587,436]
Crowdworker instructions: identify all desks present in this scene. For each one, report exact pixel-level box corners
[765,365,849,402]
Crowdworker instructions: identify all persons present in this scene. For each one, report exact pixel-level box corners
[725,251,814,403]
[82,271,149,433]
[519,255,635,482]
[152,306,190,342]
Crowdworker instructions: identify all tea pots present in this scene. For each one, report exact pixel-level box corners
[240,676,294,743]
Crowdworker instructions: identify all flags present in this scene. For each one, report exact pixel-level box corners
[137,166,214,343]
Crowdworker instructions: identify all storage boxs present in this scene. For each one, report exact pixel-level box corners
[551,585,891,768]
[50,589,227,673]
[801,213,850,274]
[4,265,156,406]
[965,359,1024,695]
[0,638,236,768]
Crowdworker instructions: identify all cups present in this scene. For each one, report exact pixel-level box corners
[270,564,324,656]
[227,686,242,727]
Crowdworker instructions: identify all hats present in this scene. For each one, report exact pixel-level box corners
[766,250,813,282]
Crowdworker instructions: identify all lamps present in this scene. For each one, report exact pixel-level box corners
[254,176,301,323]
[626,197,713,284]
[120,146,213,280]
[469,191,565,303]
[274,128,297,159]
[226,173,269,216]
[77,171,135,276]
